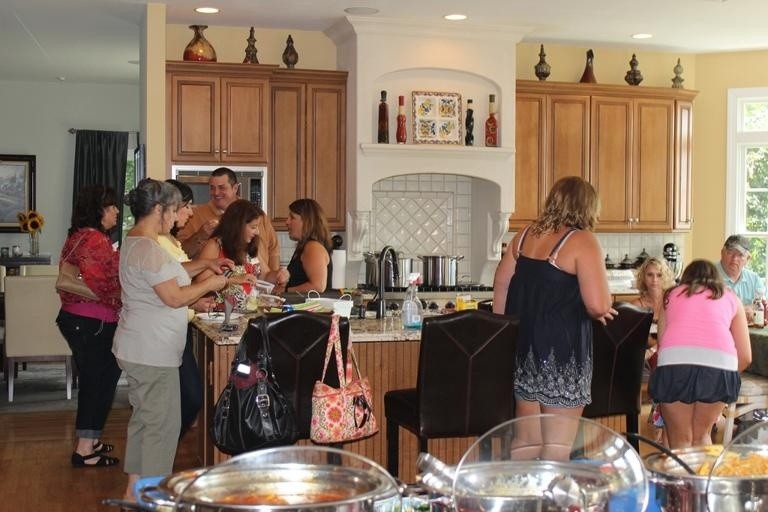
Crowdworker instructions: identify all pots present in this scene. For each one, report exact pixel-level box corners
[364,250,462,287]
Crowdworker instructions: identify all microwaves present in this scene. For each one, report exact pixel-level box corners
[172,165,268,221]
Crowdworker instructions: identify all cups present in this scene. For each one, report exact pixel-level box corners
[12,242,24,257]
[1,247,9,258]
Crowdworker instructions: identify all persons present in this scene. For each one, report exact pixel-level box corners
[110,178,235,510]
[488,177,620,461]
[172,166,280,273]
[710,233,766,307]
[53,186,125,468]
[150,179,202,445]
[270,198,334,294]
[190,198,290,310]
[627,256,676,321]
[647,258,753,451]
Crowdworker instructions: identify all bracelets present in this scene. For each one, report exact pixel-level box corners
[285,285,289,292]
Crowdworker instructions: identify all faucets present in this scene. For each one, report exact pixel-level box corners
[374,243,401,319]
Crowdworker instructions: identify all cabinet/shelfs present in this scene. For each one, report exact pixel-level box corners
[515,94,672,234]
[674,99,693,230]
[164,72,277,167]
[269,80,348,232]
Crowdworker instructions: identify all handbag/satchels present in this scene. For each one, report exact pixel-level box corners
[210,315,298,455]
[308,313,379,445]
[54,261,101,302]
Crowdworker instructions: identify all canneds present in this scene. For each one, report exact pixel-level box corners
[12,246,20,258]
[461,300,478,311]
[1,247,9,259]
[455,292,471,309]
[282,306,293,312]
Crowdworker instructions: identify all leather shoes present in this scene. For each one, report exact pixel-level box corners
[93,441,114,453]
[71,450,119,468]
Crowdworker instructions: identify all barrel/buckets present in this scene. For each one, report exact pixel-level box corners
[642,444,766,511]
[420,452,613,512]
[157,464,409,511]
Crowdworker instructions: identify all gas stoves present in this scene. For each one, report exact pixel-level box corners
[359,282,495,296]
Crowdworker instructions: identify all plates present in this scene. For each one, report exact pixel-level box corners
[196,312,244,322]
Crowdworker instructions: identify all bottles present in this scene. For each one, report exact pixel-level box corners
[377,89,409,144]
[536,43,684,88]
[465,93,498,146]
[183,22,299,69]
[603,248,650,269]
[753,293,768,328]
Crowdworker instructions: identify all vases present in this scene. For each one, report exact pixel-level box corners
[183,21,217,64]
[16,209,46,236]
[30,232,41,258]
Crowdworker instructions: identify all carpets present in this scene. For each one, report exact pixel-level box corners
[3,359,128,414]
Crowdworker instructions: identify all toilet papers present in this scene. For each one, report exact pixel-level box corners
[330,247,347,291]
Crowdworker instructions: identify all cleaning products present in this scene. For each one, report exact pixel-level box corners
[399,273,425,327]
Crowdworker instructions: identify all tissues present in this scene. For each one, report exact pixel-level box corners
[364,310,377,319]
[456,293,480,312]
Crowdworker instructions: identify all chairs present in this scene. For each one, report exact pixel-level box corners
[0,264,76,405]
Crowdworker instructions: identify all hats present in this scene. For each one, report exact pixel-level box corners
[723,235,752,256]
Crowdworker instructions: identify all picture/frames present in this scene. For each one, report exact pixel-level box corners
[411,90,464,144]
[0,154,38,232]
